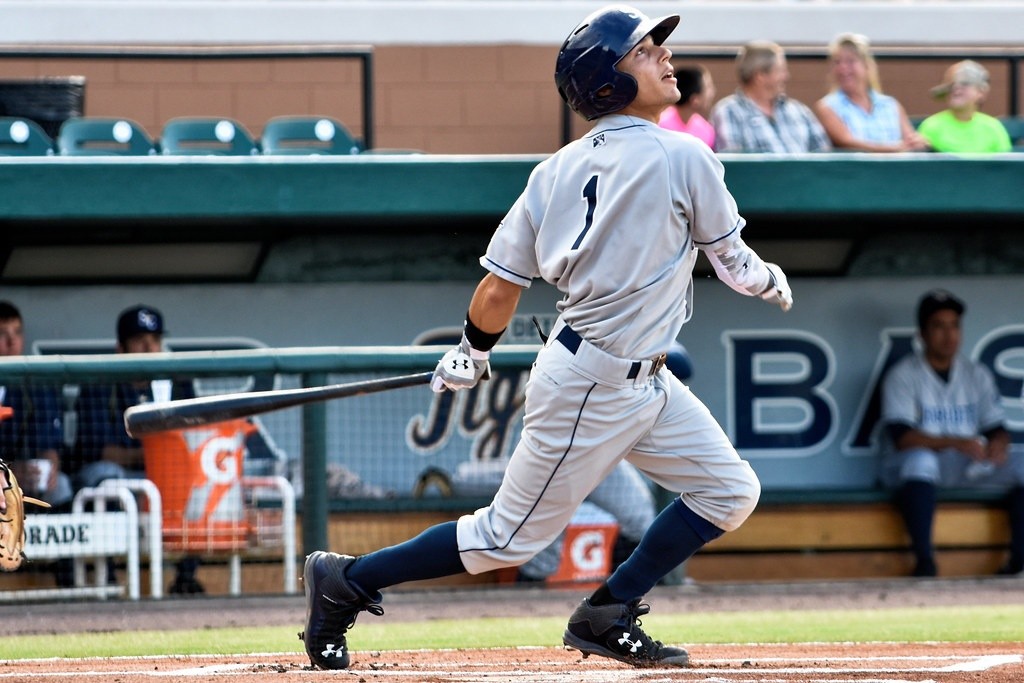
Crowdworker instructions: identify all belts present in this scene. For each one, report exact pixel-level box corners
[556,326,667,381]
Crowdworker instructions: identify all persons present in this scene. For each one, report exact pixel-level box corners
[303,4,793,670]
[2,292,80,609]
[881,292,1024,578]
[67,302,213,601]
[652,27,1014,166]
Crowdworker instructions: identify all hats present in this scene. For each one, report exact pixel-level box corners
[916,288,965,319]
[929,59,994,100]
[117,303,169,339]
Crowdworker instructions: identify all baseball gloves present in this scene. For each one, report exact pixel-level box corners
[0,455,27,572]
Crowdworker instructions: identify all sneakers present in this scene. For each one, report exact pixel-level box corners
[563,598,692,668]
[296,552,383,669]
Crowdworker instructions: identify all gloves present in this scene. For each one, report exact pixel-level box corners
[759,261,794,315]
[429,311,507,394]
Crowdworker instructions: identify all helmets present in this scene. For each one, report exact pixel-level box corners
[556,6,681,124]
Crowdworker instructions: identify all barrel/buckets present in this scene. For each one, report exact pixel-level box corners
[138,421,257,551]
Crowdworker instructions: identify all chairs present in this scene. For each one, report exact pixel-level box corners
[258,114,362,153]
[906,110,932,128]
[996,112,1024,150]
[56,116,156,156]
[158,114,257,155]
[0,114,54,156]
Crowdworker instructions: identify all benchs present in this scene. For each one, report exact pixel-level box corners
[0,484,1013,599]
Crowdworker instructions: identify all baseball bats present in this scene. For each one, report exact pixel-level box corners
[123,358,493,440]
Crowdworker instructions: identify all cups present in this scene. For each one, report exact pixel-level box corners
[28,459,51,491]
